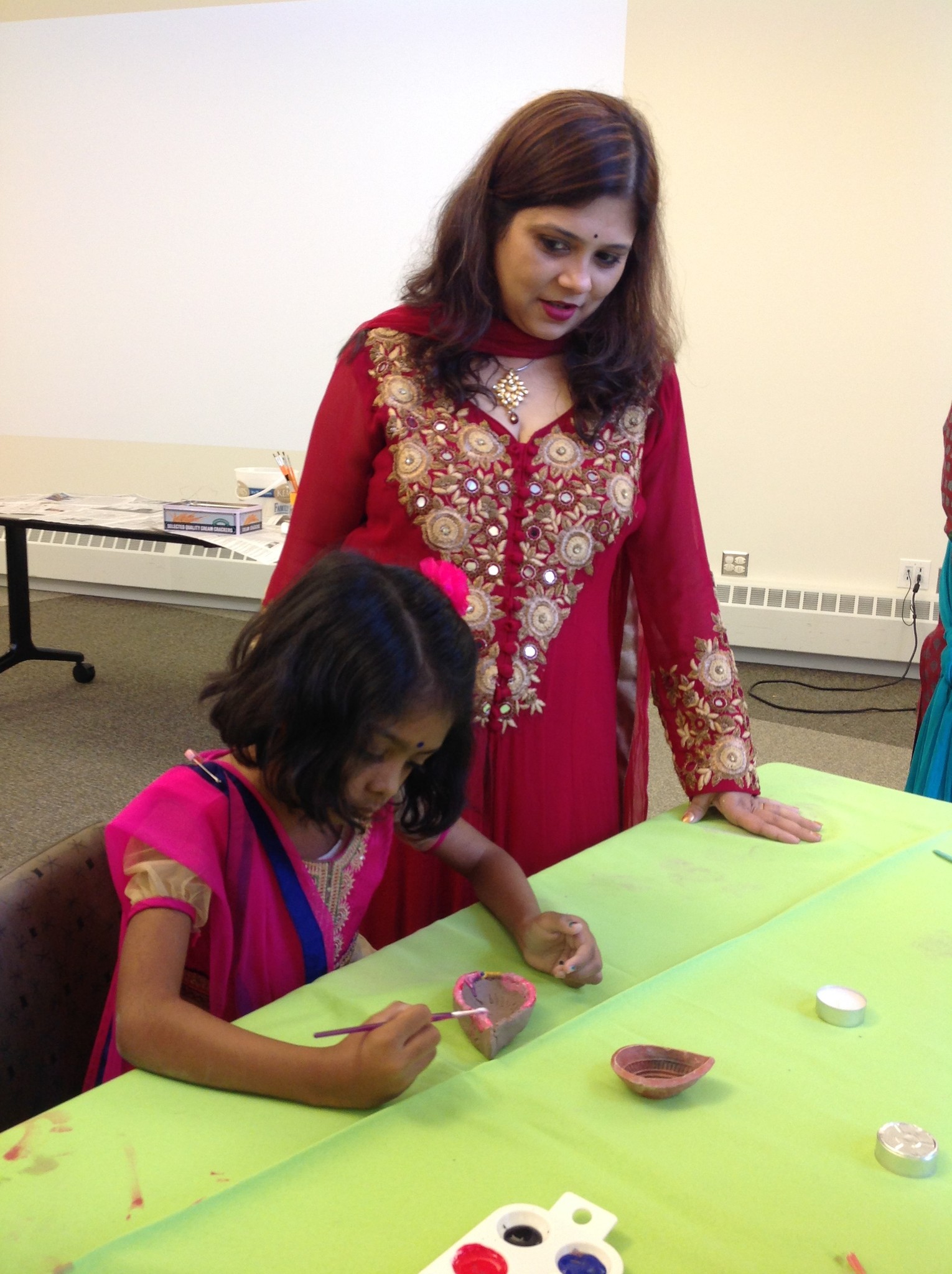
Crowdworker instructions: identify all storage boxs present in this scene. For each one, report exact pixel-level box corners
[163,502,263,534]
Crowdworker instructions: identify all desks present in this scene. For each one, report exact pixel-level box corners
[0,500,286,683]
[0,763,952,1273]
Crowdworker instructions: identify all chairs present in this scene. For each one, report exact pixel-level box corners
[0,821,114,1131]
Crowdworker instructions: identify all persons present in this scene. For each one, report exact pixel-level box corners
[82,550,604,1110]
[264,91,823,951]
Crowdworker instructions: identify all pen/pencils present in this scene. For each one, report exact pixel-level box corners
[933,849,952,863]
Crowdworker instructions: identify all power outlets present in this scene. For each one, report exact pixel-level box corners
[722,551,748,577]
[896,558,931,591]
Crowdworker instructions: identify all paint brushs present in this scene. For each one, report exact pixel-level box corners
[315,1007,488,1038]
[272,451,297,493]
